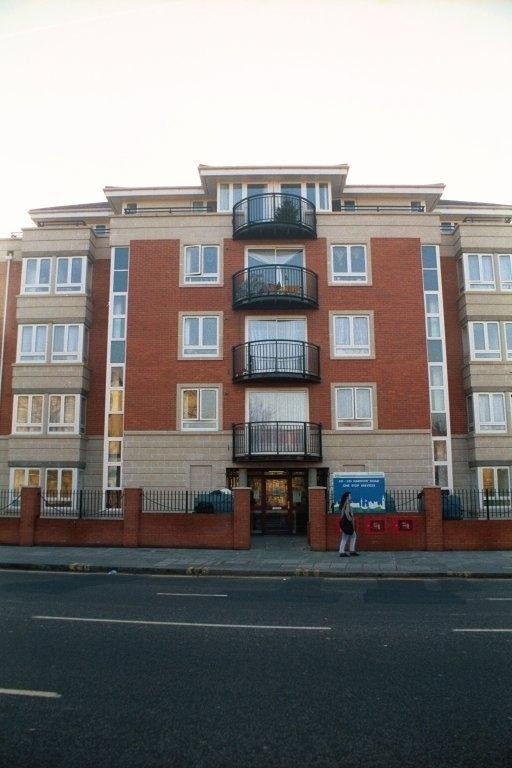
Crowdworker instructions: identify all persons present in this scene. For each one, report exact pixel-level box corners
[338,492,360,557]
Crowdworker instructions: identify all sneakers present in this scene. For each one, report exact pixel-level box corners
[340,553,349,557]
[350,551,359,556]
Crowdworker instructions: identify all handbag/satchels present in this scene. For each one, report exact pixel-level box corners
[339,513,354,535]
